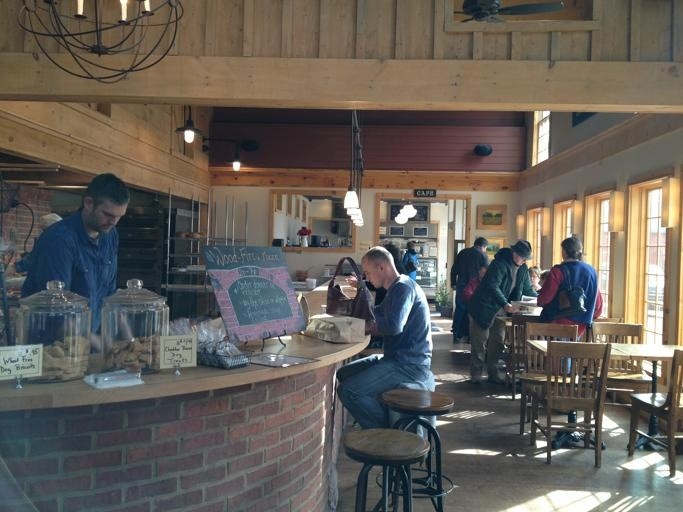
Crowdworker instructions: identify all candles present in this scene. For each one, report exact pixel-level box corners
[75,0,154,20]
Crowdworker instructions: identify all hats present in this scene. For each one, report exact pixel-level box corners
[510,241,533,260]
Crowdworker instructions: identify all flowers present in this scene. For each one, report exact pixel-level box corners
[296,226,311,236]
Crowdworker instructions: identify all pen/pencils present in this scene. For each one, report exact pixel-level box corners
[287,238,289,243]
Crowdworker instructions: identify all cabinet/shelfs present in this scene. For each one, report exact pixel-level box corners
[413,257,437,300]
[160,235,247,294]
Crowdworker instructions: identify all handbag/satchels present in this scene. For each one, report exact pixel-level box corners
[539,287,586,321]
[325,257,375,320]
[405,261,416,274]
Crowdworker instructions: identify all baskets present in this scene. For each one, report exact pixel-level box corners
[197,351,254,370]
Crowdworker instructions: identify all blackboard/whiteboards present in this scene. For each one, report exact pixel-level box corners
[388,203,430,223]
[413,226,428,237]
[202,246,306,343]
[389,226,405,237]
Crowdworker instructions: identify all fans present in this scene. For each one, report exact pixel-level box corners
[453,0,567,25]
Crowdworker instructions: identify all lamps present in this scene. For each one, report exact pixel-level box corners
[608,191,624,232]
[174,105,203,144]
[569,200,582,235]
[394,213,408,224]
[514,212,524,239]
[658,177,679,228]
[201,136,246,171]
[399,198,418,218]
[539,208,550,236]
[343,107,367,227]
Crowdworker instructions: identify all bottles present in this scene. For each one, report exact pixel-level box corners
[320,303,327,314]
[99,279,170,376]
[284,234,311,248]
[320,233,352,246]
[13,279,91,385]
[323,268,328,276]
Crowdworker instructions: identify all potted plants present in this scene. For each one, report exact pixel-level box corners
[438,280,452,318]
[432,282,440,311]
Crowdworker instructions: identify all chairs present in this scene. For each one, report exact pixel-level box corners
[494,312,682,478]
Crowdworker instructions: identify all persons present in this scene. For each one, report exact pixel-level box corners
[451,237,490,341]
[537,236,604,376]
[22,172,133,352]
[337,245,433,427]
[538,270,551,290]
[462,264,488,302]
[466,240,540,385]
[367,240,408,345]
[529,266,542,292]
[8,213,62,275]
[403,242,417,282]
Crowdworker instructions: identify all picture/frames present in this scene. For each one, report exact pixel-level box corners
[485,236,507,265]
[476,203,508,231]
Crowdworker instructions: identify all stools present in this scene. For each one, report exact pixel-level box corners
[375,387,458,511]
[341,427,431,512]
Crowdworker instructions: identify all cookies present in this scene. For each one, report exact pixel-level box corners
[101,335,161,373]
[27,334,92,383]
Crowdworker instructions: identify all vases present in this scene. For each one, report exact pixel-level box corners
[300,235,308,247]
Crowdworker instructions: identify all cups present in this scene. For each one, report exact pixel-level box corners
[305,279,317,294]
[294,269,308,283]
